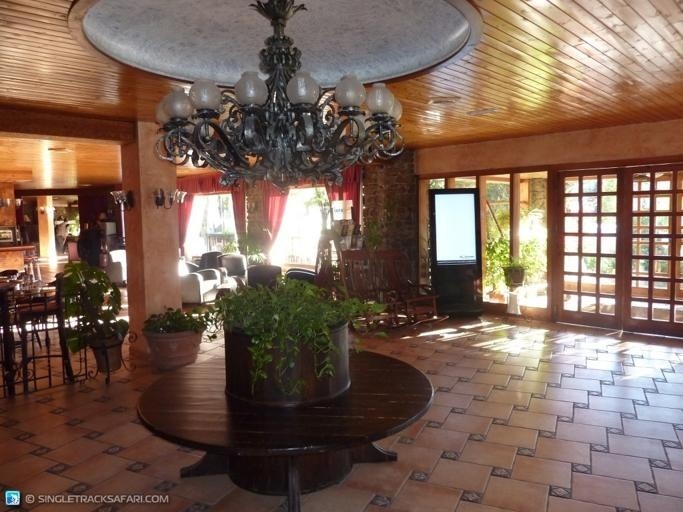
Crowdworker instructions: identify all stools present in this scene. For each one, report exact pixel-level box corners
[24,255,42,283]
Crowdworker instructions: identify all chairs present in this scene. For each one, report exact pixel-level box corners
[373,248,446,324]
[66,241,82,265]
[340,247,401,331]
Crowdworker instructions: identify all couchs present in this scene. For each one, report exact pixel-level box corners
[106,247,281,305]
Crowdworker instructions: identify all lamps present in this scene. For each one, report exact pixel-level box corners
[149,0,403,191]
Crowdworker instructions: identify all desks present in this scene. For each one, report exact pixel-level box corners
[134,351,435,512]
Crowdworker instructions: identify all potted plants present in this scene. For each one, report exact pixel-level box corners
[139,308,202,370]
[61,263,124,372]
[480,235,535,290]
[185,275,387,404]
